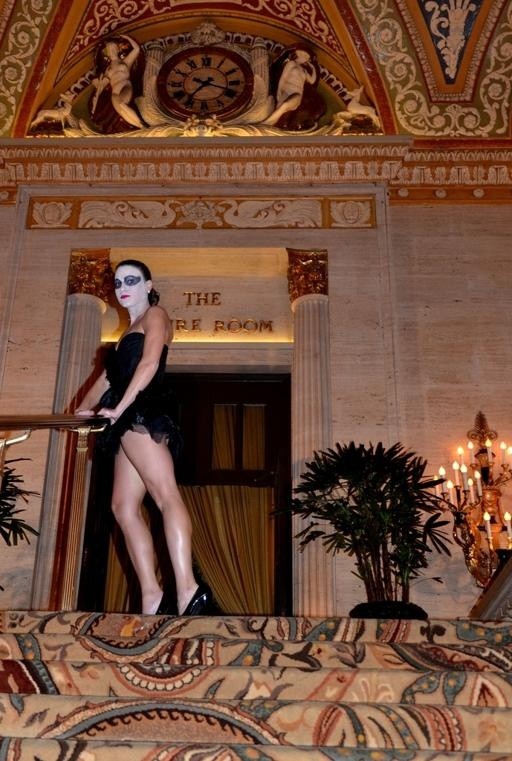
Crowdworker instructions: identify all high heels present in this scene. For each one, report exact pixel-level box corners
[157,592,176,614]
[182,583,212,615]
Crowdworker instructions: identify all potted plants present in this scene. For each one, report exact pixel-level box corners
[268,439,468,620]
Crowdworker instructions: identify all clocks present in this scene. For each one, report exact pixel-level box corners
[156,44,255,123]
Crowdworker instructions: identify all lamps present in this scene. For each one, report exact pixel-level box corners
[432,410,512,588]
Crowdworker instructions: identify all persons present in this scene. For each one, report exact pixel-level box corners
[93,34,145,129]
[72,259,212,617]
[252,50,316,125]
